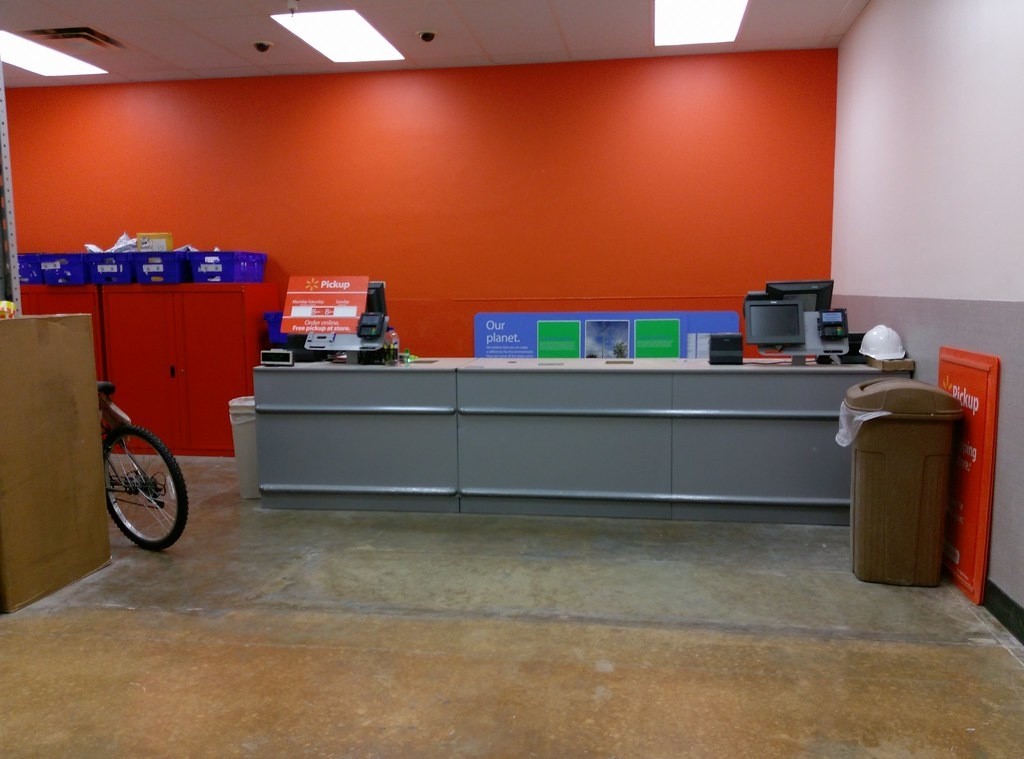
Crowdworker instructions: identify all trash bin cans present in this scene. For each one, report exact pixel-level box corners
[229,396,259,499]
[844,375,968,592]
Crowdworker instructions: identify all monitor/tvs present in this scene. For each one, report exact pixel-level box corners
[744,280,834,350]
[366,281,387,316]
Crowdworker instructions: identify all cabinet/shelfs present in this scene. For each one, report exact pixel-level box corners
[18,284,107,455]
[103,281,284,457]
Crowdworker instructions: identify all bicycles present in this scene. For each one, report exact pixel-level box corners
[96,381,188,550]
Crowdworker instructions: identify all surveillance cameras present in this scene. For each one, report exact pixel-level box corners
[252,41,273,52]
[416,29,437,42]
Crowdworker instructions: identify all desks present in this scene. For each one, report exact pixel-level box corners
[251,355,915,525]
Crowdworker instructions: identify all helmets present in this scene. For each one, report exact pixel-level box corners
[859,325,905,360]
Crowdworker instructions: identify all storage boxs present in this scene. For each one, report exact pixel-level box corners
[133,251,188,283]
[37,253,86,285]
[187,251,267,282]
[264,312,288,342]
[17,254,44,284]
[87,253,134,283]
